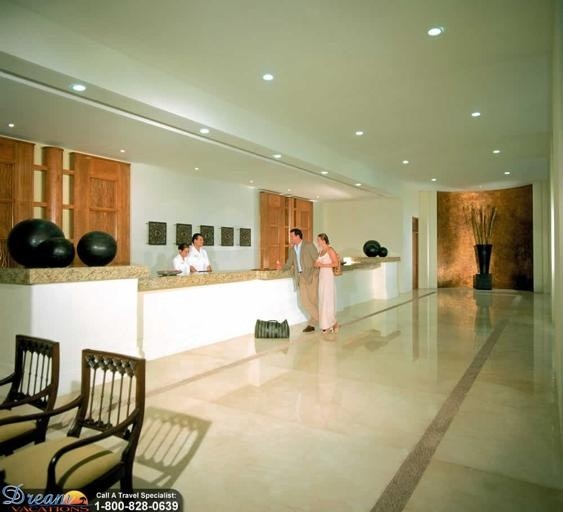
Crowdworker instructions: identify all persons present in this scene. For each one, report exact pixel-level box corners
[187,233,211,272]
[313,233,338,334]
[282,229,319,332]
[172,243,189,275]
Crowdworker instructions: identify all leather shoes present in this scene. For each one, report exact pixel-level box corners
[303,325,314,332]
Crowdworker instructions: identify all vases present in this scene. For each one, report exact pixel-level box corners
[475,305,492,333]
[476,244,492,274]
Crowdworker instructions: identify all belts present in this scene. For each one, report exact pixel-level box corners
[298,271,303,273]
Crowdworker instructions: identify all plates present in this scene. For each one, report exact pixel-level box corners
[156,269,182,276]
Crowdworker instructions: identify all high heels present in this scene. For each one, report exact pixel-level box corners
[329,323,339,334]
[321,329,328,334]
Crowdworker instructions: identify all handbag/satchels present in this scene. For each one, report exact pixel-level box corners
[255,319,289,338]
[334,254,342,276]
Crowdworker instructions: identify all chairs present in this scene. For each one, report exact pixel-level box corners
[0,335,59,457]
[0,349,145,512]
[101,406,212,512]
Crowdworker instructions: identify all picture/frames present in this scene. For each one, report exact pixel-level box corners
[176,223,191,246]
[240,228,250,246]
[200,226,214,245]
[221,227,233,246]
[148,222,165,244]
